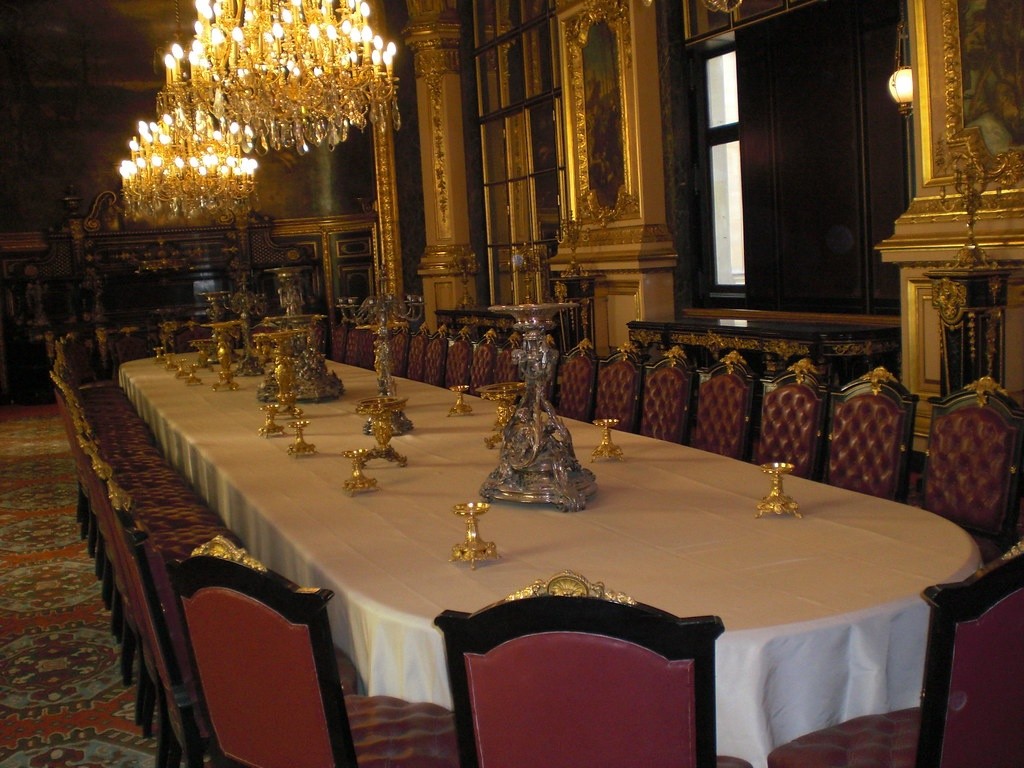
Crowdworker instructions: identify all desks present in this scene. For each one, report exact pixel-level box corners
[118,351,982,766]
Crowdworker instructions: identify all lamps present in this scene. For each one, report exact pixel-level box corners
[888,1,914,119]
[117,0,395,227]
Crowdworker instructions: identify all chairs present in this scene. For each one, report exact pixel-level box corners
[49,310,1024,766]
